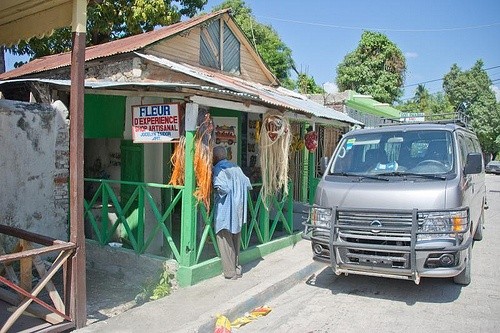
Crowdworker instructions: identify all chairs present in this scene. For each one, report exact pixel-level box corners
[365,148,389,167]
[425,142,446,160]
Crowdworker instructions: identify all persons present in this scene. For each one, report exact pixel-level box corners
[212,146,253,280]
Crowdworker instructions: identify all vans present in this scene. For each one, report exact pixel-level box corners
[301,110,489,286]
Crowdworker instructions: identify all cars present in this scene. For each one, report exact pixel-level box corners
[485,160,500,174]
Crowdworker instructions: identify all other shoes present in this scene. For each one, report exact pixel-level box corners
[222,272,242,280]
